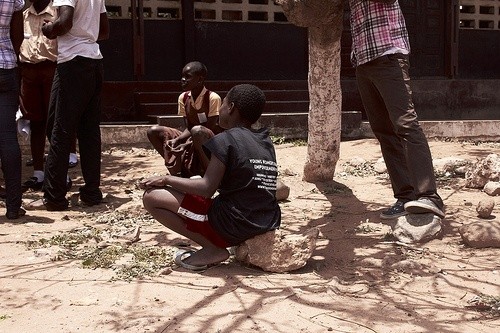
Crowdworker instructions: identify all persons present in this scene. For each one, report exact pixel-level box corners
[139,61,282,271]
[0,0,110,220]
[351,0,447,220]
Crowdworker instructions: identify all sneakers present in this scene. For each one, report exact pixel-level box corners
[380,197,445,219]
[6,208,26,219]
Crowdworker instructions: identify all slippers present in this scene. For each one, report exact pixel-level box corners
[174,251,207,271]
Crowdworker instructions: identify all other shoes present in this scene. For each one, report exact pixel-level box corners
[22,177,44,192]
[24,198,46,210]
[68,159,78,168]
[0,185,7,199]
